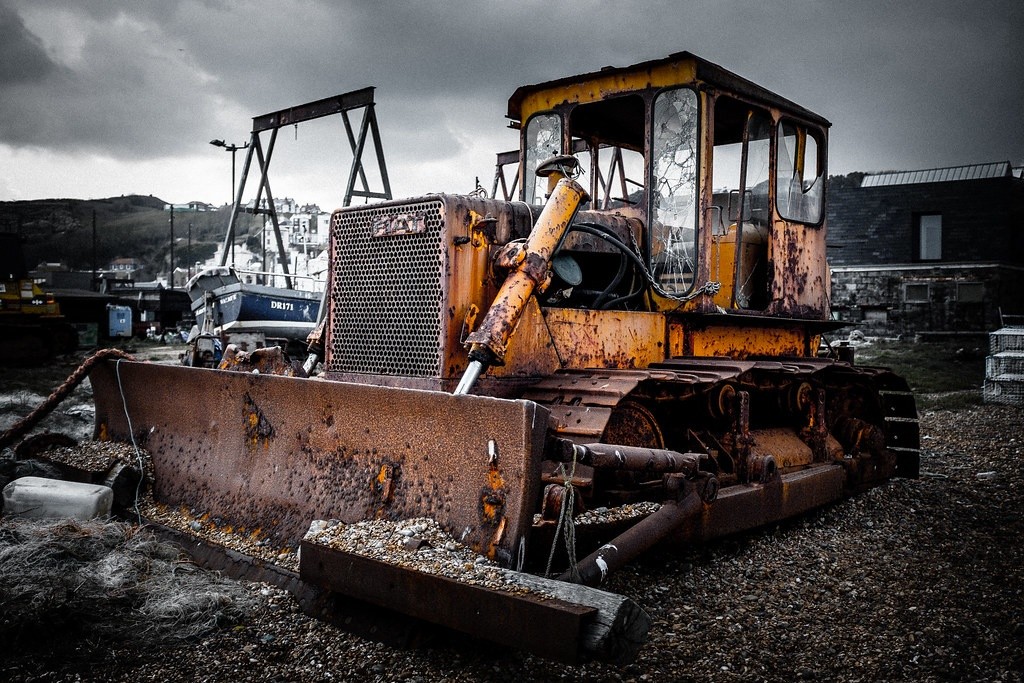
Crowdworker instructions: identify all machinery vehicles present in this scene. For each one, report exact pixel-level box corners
[0,51,921,666]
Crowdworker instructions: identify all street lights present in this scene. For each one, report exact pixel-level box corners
[148,194,197,288]
[211,138,250,270]
